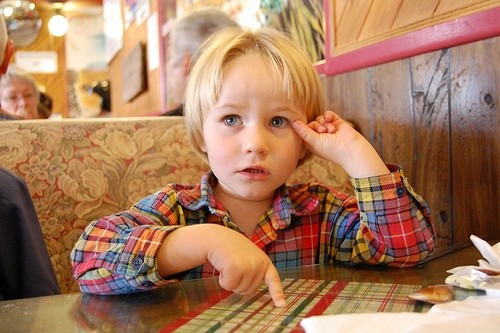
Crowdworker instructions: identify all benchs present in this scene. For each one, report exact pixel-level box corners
[0,117,367,292]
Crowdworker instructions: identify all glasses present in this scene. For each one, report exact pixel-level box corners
[0,92,37,105]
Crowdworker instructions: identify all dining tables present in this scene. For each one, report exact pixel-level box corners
[0,235,500,333]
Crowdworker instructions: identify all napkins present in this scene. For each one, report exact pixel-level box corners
[471,232,500,273]
[300,295,500,333]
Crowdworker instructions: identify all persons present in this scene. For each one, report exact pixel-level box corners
[72,28,440,309]
[37,93,53,120]
[0,71,42,121]
[0,12,62,301]
[147,8,241,118]
